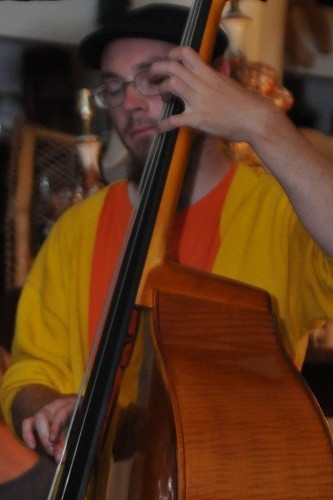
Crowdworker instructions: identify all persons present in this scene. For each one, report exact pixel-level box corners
[0,3,333,500]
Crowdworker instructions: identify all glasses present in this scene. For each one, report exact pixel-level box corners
[88,71,170,109]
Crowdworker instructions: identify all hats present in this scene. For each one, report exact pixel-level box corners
[78,3,229,70]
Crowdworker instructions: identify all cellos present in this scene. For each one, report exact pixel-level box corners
[46,0,333,500]
